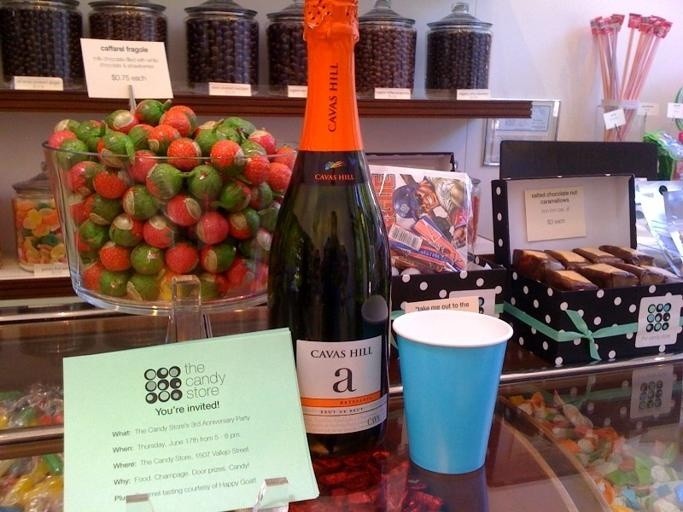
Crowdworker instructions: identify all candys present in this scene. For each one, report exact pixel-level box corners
[11,194,68,267]
[1,390,63,429]
[1,454,64,512]
[509,390,683,512]
[48,99,298,302]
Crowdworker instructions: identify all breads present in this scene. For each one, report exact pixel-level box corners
[513,245,668,291]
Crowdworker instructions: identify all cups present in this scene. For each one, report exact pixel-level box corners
[392,309,514,475]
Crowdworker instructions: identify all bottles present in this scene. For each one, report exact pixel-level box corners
[267,0,392,457]
[267,0,307,91]
[425,1,495,90]
[11,161,67,273]
[183,1,261,92]
[1,1,84,91]
[87,0,168,67]
[353,0,418,90]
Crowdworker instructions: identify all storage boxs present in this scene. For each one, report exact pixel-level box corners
[492,173,683,369]
[364,152,506,369]
